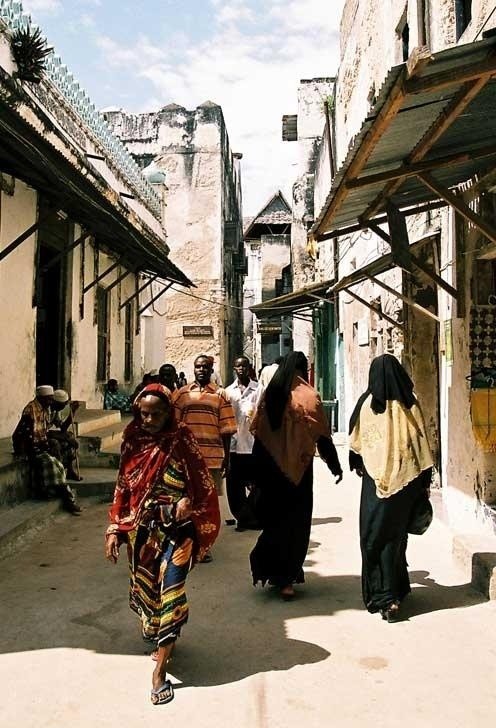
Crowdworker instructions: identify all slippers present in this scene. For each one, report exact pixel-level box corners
[149,646,173,662]
[151,681,173,704]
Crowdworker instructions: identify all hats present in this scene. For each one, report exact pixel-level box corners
[37,385,54,395]
[54,390,68,402]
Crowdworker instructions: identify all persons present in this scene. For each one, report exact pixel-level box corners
[349,356,436,623]
[9,384,81,517]
[247,350,341,599]
[103,350,295,531]
[99,384,220,703]
[46,388,82,481]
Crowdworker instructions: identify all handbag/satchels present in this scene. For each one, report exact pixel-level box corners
[237,487,261,530]
[407,496,432,535]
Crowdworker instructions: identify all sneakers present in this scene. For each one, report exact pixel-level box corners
[67,474,84,481]
[268,587,280,595]
[61,502,83,514]
[280,593,296,602]
[387,612,399,622]
[379,608,389,620]
